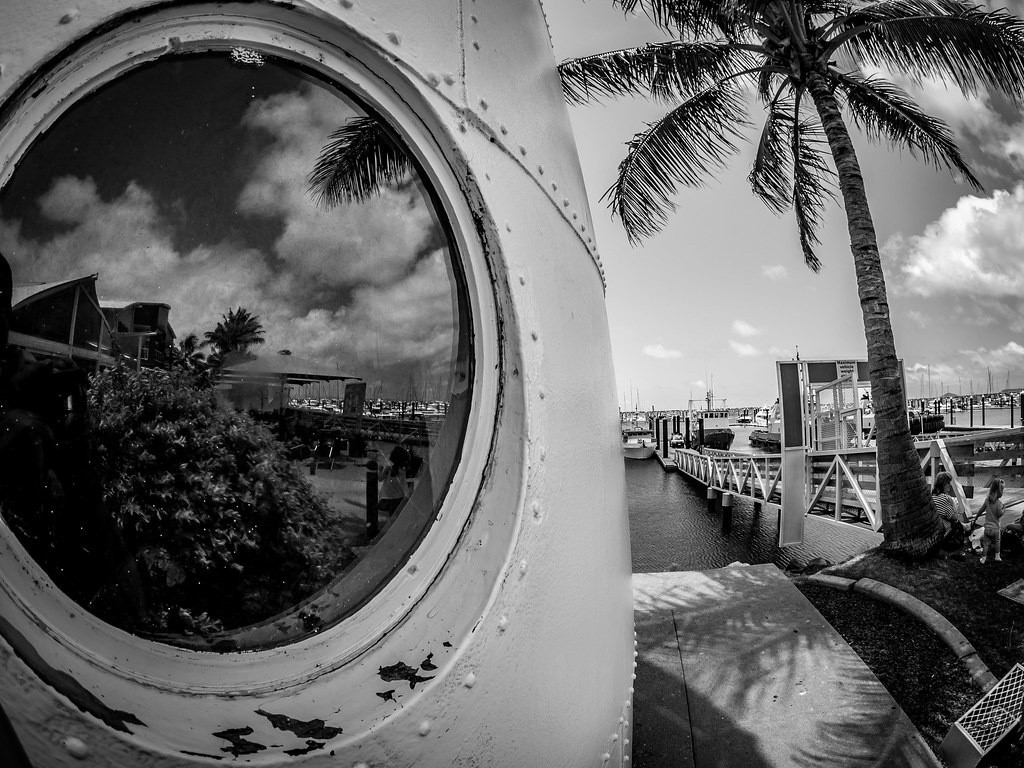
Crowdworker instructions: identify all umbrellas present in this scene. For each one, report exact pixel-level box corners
[213,349,361,415]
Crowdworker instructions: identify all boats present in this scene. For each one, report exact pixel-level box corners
[619,407,684,460]
[683,389,735,451]
[909,363,1024,417]
[737,407,752,422]
[748,351,823,452]
[832,384,946,437]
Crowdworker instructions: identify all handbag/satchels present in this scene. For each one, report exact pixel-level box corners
[939,515,966,535]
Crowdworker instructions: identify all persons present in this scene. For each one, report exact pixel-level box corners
[932,472,971,532]
[861,394,869,407]
[974,479,1006,563]
[378,447,407,513]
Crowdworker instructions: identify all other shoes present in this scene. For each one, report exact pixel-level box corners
[980,556,986,565]
[994,552,1003,562]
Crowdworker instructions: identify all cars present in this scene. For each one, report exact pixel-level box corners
[669,434,684,448]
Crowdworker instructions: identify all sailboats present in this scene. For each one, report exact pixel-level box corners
[284,370,450,421]
